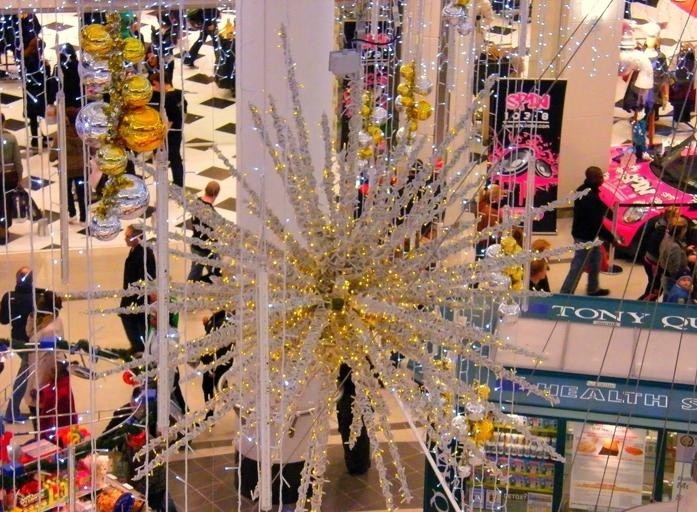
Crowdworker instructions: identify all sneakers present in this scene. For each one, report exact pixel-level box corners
[31,136,53,147]
[186,63,199,69]
[67,216,84,226]
[590,289,610,296]
[6,413,27,423]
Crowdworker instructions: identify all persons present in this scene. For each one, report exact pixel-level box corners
[0,264,63,425]
[185,180,226,282]
[20,288,69,436]
[392,155,446,251]
[120,221,247,456]
[100,387,177,512]
[638,204,697,305]
[1,1,234,245]
[557,165,621,299]
[473,192,553,293]
[21,346,83,437]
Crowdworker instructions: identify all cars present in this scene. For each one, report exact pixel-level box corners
[594,131,696,266]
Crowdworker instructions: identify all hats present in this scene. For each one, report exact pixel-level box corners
[677,269,694,283]
